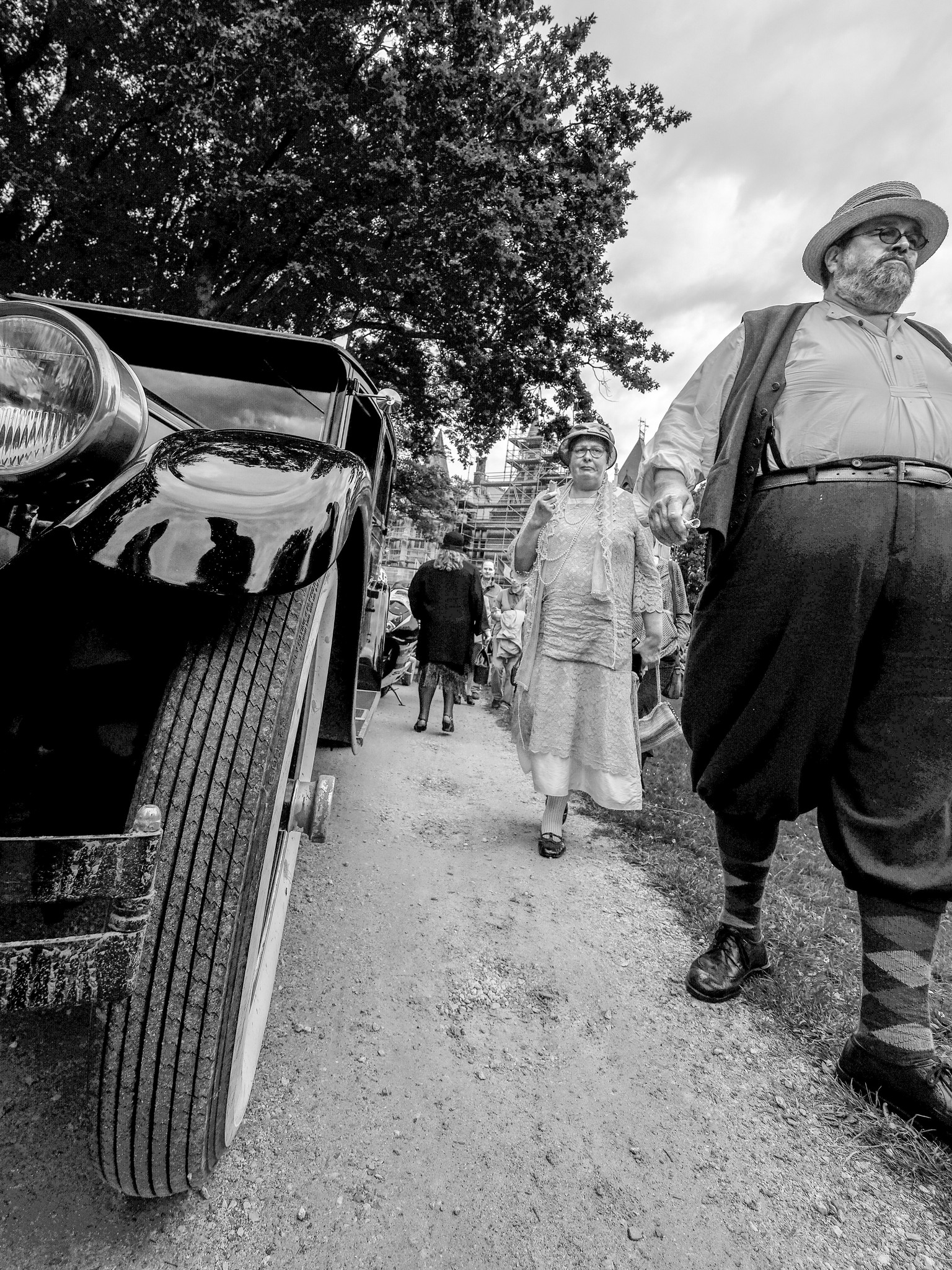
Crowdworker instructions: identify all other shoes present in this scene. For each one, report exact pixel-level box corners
[492,699,500,708]
[414,718,427,733]
[466,696,475,705]
[453,695,461,704]
[498,702,510,711]
[442,714,454,732]
[546,799,568,825]
[538,829,566,858]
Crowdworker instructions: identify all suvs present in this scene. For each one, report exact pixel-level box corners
[0,300,410,1203]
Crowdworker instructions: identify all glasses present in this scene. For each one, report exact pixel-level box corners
[844,227,930,251]
[569,446,606,458]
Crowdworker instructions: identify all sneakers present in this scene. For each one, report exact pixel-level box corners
[836,1033,952,1147]
[686,926,773,1004]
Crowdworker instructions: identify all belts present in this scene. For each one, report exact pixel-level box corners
[744,464,952,494]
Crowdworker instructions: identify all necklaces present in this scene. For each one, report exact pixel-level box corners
[535,482,600,587]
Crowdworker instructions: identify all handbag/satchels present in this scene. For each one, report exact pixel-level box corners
[661,665,685,699]
[638,663,683,753]
[510,653,523,686]
[473,648,490,685]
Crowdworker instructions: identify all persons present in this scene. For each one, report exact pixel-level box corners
[507,424,663,861]
[407,531,492,732]
[451,560,526,709]
[639,180,952,1152]
[634,538,693,799]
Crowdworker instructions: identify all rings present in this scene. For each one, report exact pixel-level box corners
[553,503,557,508]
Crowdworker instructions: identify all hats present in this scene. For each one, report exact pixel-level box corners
[440,531,465,553]
[559,422,617,470]
[801,181,949,285]
